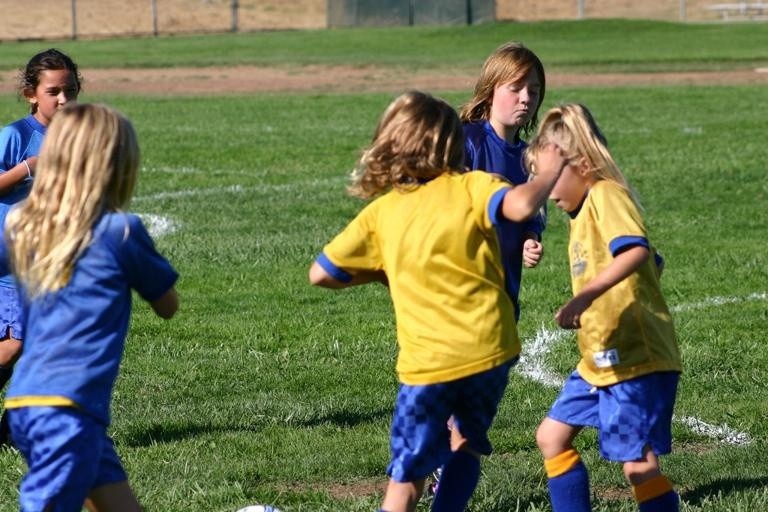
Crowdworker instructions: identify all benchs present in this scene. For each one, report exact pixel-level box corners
[711,3,768,20]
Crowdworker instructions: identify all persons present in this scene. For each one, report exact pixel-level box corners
[522,103,681,512]
[306,89,568,511]
[0,48,85,388]
[432,42,546,483]
[0,104,180,511]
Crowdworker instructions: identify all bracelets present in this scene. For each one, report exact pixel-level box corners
[22,157,33,179]
